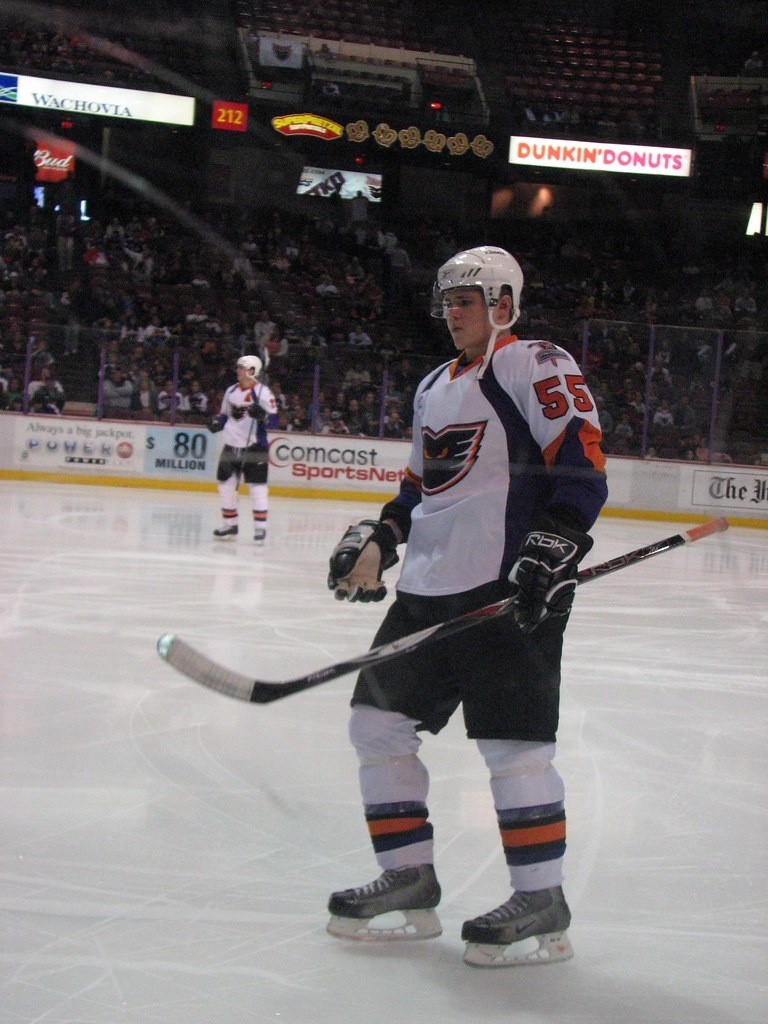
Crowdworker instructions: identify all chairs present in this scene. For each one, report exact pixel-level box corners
[235,1,668,129]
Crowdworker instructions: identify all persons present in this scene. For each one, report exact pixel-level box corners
[327,246,609,946]
[0,0,768,466]
[206,355,277,542]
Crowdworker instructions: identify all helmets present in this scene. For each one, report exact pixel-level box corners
[236,355,263,378]
[437,246,523,318]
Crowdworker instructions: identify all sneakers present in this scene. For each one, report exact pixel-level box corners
[213,525,238,539]
[461,885,574,966]
[252,528,266,545]
[326,862,443,939]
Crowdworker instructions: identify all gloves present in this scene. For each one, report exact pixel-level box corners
[207,418,223,433]
[248,404,265,420]
[510,519,594,633]
[328,519,400,603]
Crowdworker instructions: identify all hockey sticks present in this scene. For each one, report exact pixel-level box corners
[154,516,728,706]
[234,346,270,492]
